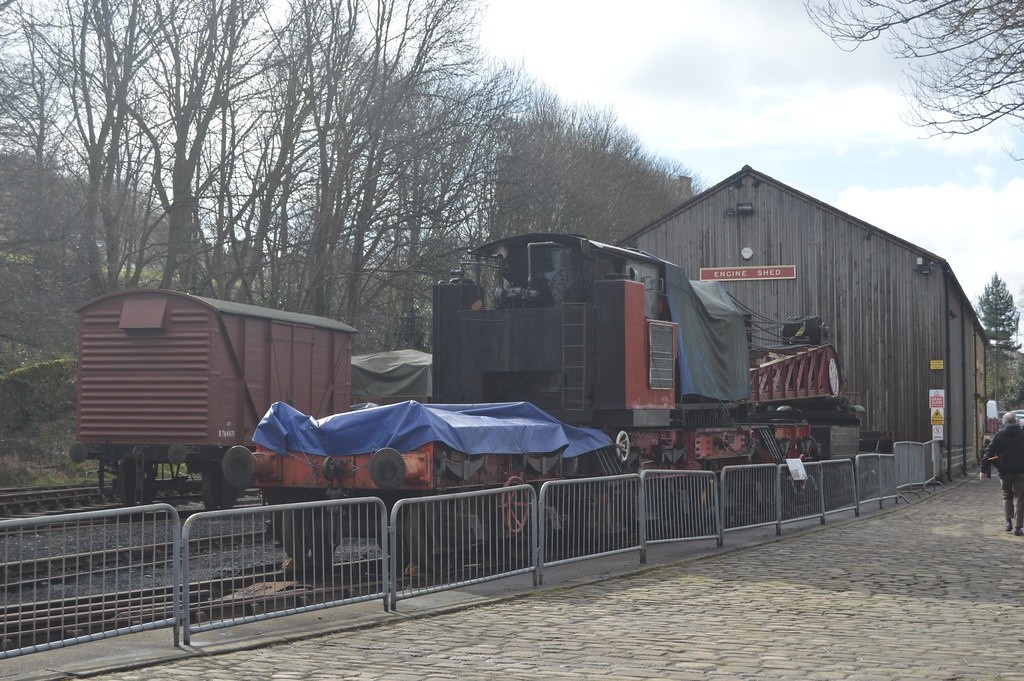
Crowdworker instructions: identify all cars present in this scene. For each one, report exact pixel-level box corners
[997,409,1024,430]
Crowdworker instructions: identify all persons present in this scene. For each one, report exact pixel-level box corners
[980,439,992,480]
[980,413,1024,536]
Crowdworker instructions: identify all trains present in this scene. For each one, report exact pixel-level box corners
[69,231,869,581]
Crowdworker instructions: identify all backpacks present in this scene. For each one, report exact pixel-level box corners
[980,445,987,458]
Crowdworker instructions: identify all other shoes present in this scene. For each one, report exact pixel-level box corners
[1005,522,1012,531]
[987,478,992,482]
[1013,528,1024,536]
